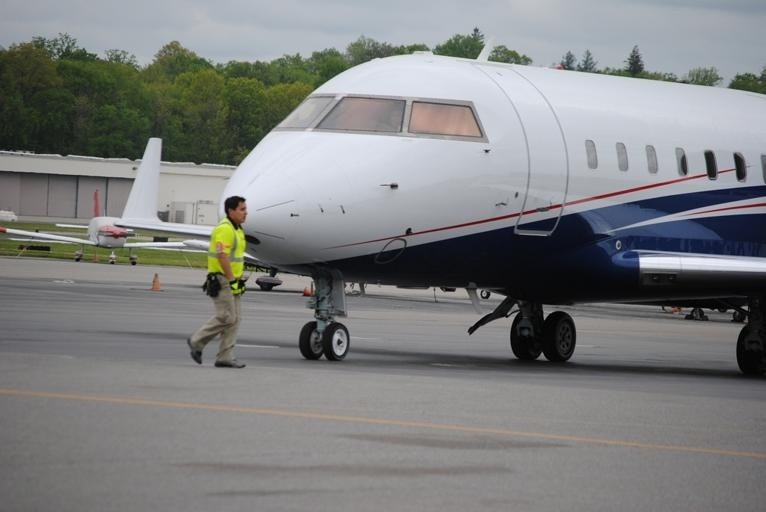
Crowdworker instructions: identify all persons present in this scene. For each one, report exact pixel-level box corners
[184,194,248,369]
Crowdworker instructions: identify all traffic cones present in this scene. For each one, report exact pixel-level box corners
[150,273,160,291]
[303,286,315,297]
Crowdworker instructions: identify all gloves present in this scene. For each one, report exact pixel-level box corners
[203,273,221,297]
[230,279,246,296]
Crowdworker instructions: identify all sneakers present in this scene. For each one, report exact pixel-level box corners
[215,359,246,368]
[187,338,202,364]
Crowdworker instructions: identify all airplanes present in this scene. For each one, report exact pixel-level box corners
[0,190,208,268]
[111,40,766,378]
